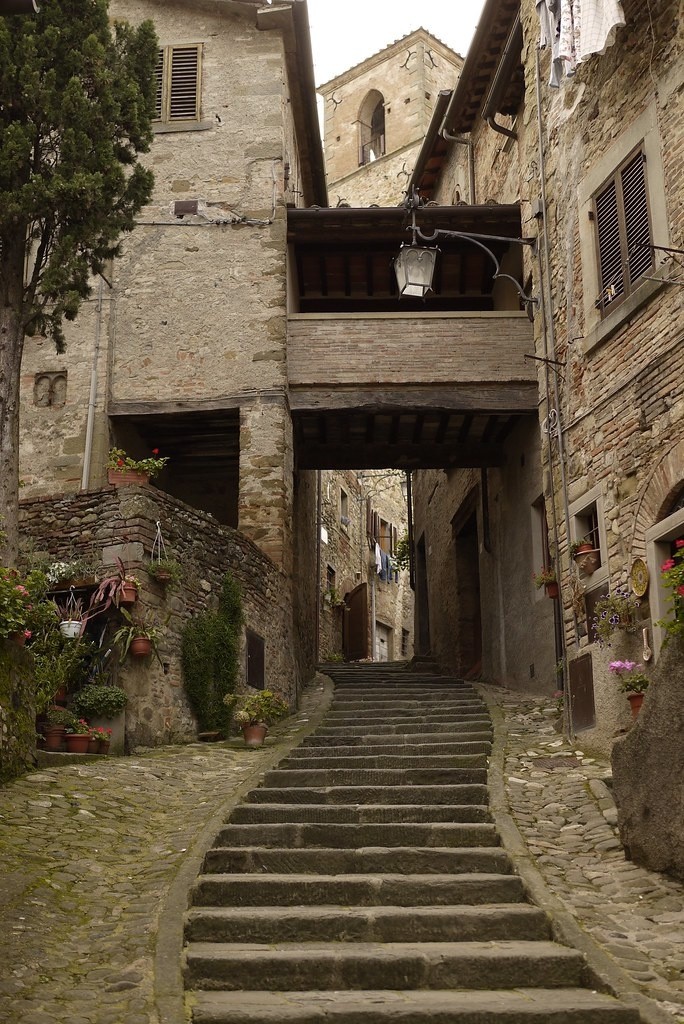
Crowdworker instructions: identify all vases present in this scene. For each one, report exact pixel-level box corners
[572,548,601,576]
[118,581,138,604]
[240,722,269,746]
[545,582,560,599]
[52,685,66,701]
[8,629,27,646]
[65,734,110,753]
[626,692,645,724]
[106,467,150,485]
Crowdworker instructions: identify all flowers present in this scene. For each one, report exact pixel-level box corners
[68,717,113,741]
[607,658,649,694]
[223,683,290,726]
[590,587,641,648]
[81,555,143,623]
[102,446,170,478]
[531,566,557,591]
[0,566,58,639]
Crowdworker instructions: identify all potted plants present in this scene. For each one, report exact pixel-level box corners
[113,605,173,669]
[568,536,593,564]
[42,708,75,751]
[142,520,181,584]
[322,588,332,601]
[52,585,84,638]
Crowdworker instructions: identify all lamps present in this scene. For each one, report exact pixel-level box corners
[389,183,539,306]
